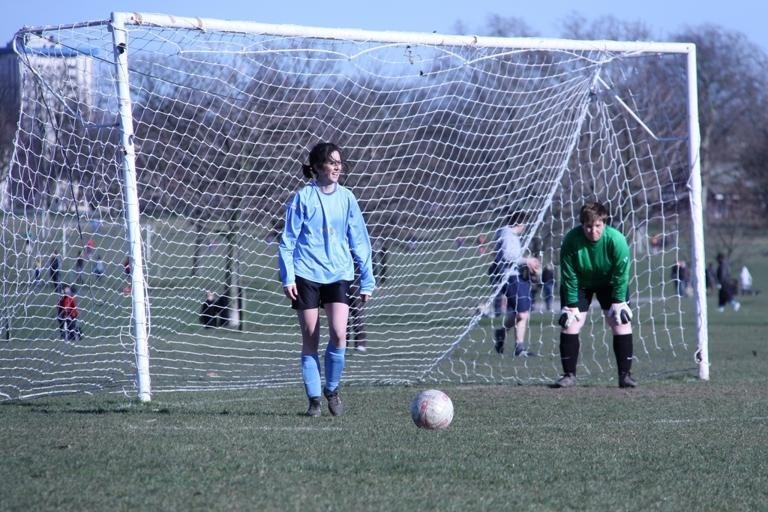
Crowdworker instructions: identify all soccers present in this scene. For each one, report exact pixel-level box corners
[411,389,454,429]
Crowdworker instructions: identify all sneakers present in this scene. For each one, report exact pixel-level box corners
[515,349,534,357]
[305,396,322,418]
[554,372,577,388]
[618,371,637,388]
[494,327,509,356]
[323,386,343,416]
[715,302,741,313]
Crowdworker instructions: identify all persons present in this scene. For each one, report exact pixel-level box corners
[670,252,754,312]
[199,286,243,329]
[24,219,130,342]
[488,201,639,388]
[279,142,374,417]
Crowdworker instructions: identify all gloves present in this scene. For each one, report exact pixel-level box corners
[556,305,581,330]
[607,302,633,326]
